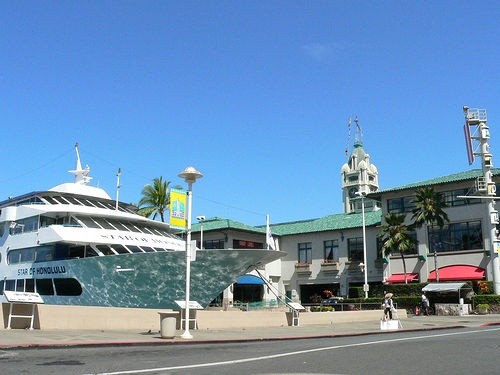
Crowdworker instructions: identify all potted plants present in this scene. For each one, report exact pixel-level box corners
[476,304,489,314]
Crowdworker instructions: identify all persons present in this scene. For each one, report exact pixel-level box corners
[422,294,429,315]
[384,293,393,320]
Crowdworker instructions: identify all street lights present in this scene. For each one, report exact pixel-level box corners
[178,166,204,340]
[354,190,369,298]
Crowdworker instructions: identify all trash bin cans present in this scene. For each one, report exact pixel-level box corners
[158,311,178,338]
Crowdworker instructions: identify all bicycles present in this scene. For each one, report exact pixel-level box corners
[413,302,434,315]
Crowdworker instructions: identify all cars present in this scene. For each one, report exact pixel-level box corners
[322,296,345,309]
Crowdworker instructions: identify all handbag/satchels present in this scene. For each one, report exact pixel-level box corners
[390,307,397,313]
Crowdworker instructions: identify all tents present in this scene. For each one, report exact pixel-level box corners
[422,282,473,304]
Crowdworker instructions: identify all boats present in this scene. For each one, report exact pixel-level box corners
[0,141,288,311]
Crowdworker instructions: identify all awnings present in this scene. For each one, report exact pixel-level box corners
[386,273,418,282]
[428,265,486,281]
